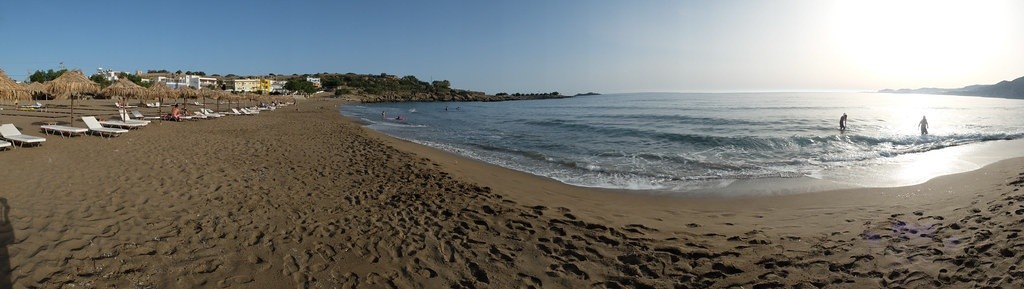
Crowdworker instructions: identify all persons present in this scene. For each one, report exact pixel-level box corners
[839,113,848,131]
[918,116,928,136]
[171,103,182,120]
[171,104,182,121]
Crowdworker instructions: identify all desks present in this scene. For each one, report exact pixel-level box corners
[98,122,148,131]
[41,125,88,139]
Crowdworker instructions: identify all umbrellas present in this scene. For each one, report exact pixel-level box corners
[0,65,295,123]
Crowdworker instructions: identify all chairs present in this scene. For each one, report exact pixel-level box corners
[0,101,292,152]
